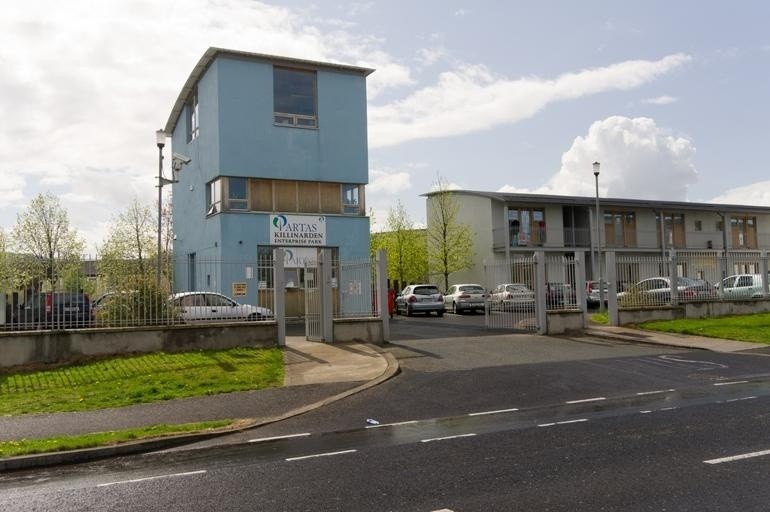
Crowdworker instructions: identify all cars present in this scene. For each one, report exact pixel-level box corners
[440,284,490,313]
[491,284,534,312]
[395,284,444,315]
[544,270,769,311]
[163,293,276,321]
[90,290,136,316]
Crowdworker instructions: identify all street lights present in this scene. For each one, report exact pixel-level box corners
[593,161,607,313]
[154,129,167,290]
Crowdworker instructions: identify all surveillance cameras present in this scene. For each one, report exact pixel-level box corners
[172,151,192,165]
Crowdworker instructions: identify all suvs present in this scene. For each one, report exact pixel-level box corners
[15,290,94,328]
